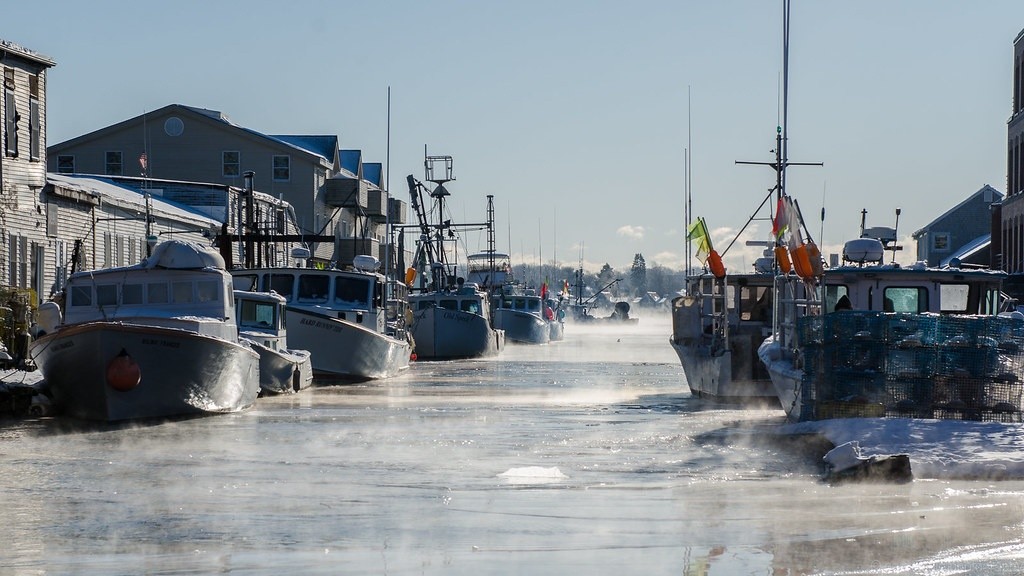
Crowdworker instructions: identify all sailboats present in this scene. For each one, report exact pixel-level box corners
[366,85,625,361]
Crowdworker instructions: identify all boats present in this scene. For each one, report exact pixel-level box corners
[208,171,418,393]
[28,110,262,416]
[669,0,1024,422]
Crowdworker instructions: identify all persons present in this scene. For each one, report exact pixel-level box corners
[404,305,413,331]
[546,306,554,321]
